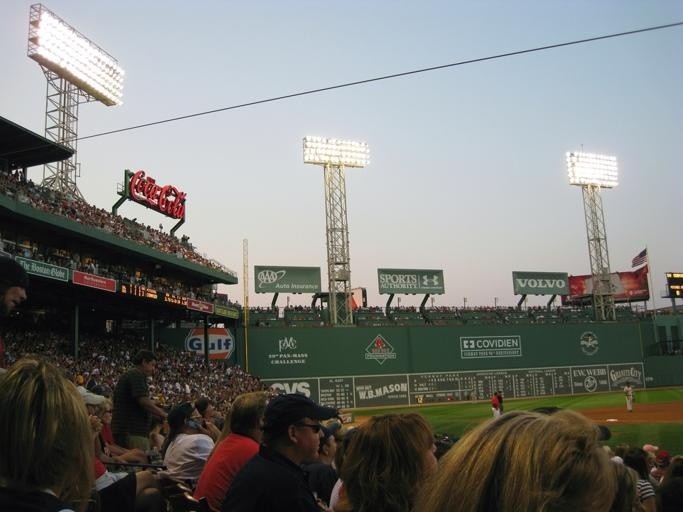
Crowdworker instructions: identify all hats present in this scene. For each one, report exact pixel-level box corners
[320,422,341,447]
[167,401,196,441]
[262,394,338,434]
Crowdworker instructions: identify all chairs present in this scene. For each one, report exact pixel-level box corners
[172,482,219,511]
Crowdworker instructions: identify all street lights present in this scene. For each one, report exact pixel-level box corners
[300,132,374,327]
[26,2,128,197]
[565,149,621,321]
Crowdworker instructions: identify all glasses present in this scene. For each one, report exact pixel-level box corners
[299,421,321,433]
